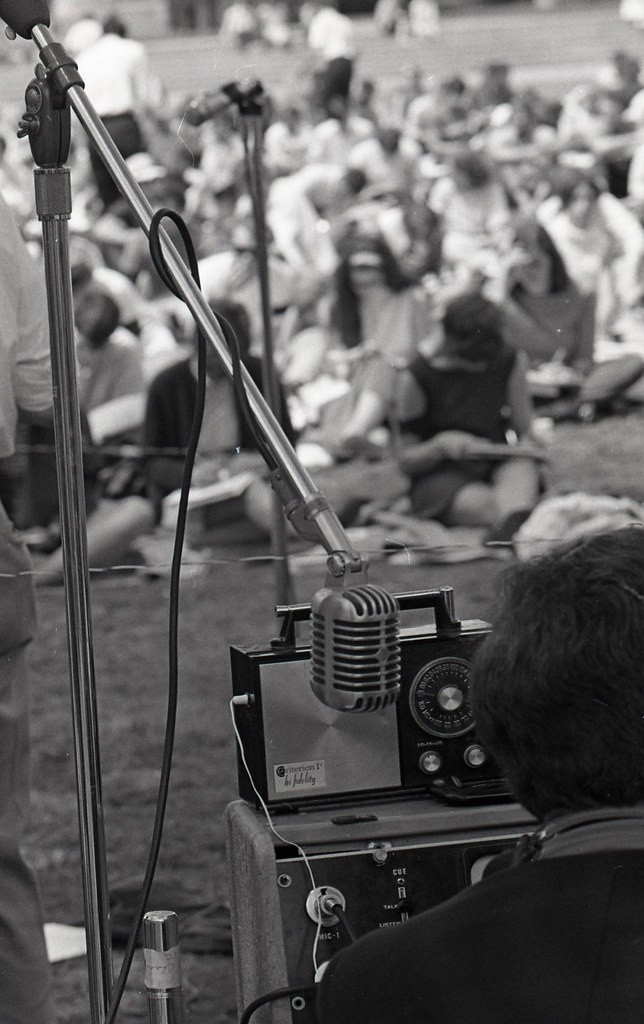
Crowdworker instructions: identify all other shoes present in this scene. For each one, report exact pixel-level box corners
[482,503,533,548]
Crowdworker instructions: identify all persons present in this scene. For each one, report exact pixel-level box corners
[1,1,644,566]
[0,507,60,1024]
[314,525,644,1024]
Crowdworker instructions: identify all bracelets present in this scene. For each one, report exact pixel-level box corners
[431,436,445,463]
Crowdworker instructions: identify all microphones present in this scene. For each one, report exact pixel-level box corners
[309,552,401,714]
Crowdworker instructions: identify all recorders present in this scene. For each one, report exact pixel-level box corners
[229,584,512,813]
